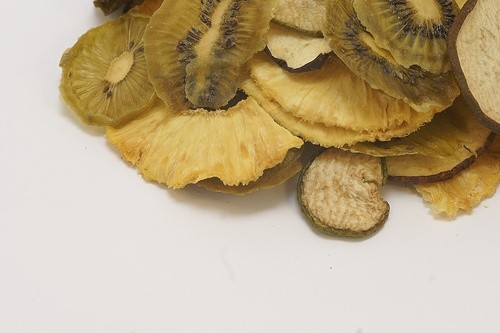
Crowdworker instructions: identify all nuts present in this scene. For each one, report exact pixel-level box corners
[59,1,500,239]
